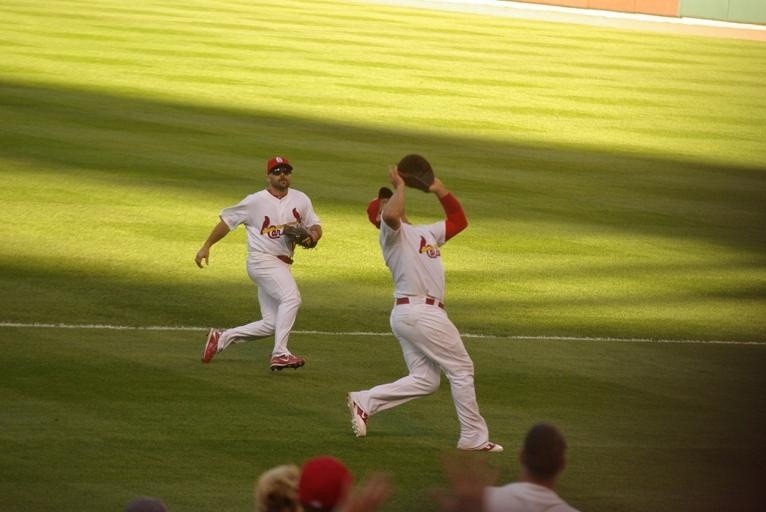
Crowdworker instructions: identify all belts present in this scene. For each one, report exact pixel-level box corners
[394,295,446,309]
[276,254,295,265]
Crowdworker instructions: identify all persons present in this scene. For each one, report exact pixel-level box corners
[484,420,583,511]
[194,155,324,373]
[251,462,299,512]
[340,446,500,511]
[343,153,507,456]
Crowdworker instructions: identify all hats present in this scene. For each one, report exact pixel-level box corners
[266,155,294,174]
[367,186,394,230]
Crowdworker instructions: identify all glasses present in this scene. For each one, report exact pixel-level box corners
[271,166,291,176]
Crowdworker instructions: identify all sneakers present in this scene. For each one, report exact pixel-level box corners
[202,326,224,363]
[457,439,508,453]
[269,352,306,372]
[346,391,368,438]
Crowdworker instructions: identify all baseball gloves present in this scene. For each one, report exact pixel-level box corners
[280,223,317,249]
[397,154,434,193]
